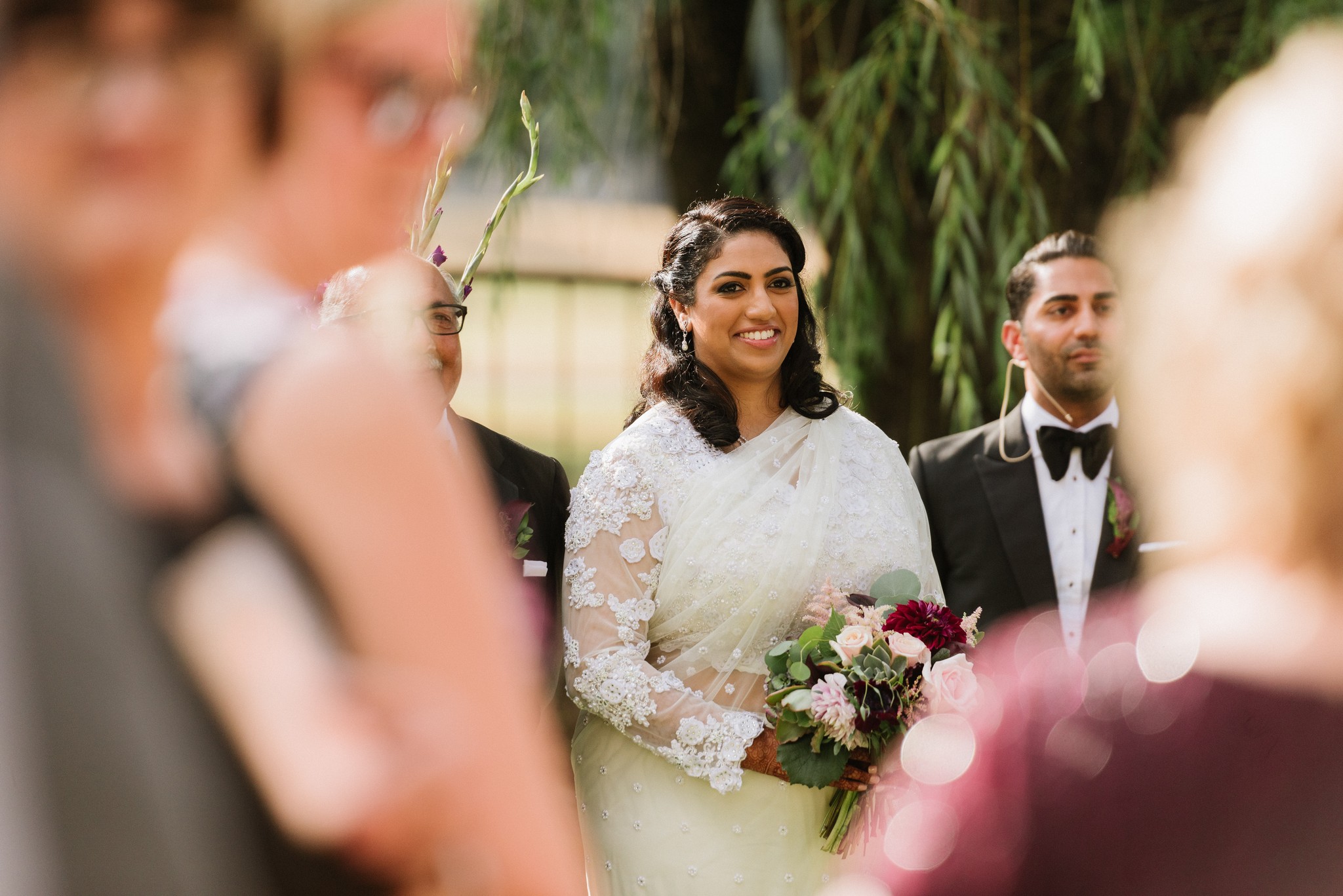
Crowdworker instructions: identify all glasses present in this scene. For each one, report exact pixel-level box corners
[417,304,468,335]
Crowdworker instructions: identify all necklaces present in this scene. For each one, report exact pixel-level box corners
[737,431,783,470]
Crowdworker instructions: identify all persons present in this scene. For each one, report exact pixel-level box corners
[910,227,1144,631]
[844,15,1340,894]
[0,0,397,896]
[158,0,582,894]
[561,196,946,895]
[328,249,569,714]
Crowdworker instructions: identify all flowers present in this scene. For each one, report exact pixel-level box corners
[497,498,536,560]
[1106,476,1140,558]
[761,570,989,860]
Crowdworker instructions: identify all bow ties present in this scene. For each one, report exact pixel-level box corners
[1035,423,1116,484]
